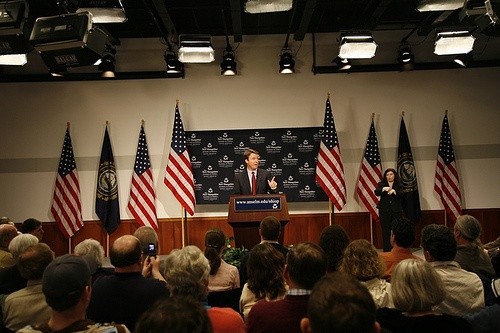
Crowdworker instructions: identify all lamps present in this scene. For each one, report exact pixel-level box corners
[280,48,296,73]
[30,12,109,72]
[62,0,130,23]
[98,44,117,71]
[331,55,351,71]
[179,33,215,64]
[163,47,182,72]
[397,38,412,65]
[221,48,237,76]
[433,26,475,56]
[338,31,377,59]
[0,2,36,64]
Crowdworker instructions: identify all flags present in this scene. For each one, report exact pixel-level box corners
[397,117,422,223]
[163,105,197,217]
[356,120,384,225]
[51,127,84,239]
[315,97,347,211]
[434,114,463,224]
[95,125,121,235]
[128,127,159,234]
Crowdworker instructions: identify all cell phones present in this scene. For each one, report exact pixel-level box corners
[147,242,156,263]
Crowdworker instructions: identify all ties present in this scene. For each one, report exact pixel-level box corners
[251,172,256,195]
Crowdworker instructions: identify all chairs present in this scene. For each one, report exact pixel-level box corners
[208,285,244,313]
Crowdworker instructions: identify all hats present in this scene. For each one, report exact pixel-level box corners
[490,278,499,297]
[41,253,98,311]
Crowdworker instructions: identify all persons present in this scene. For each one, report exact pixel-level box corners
[0,216,500,333]
[233,149,279,195]
[375,168,404,252]
